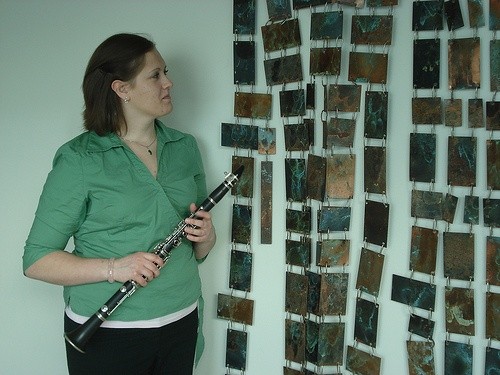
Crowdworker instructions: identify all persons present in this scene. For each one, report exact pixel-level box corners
[22,32,216,375]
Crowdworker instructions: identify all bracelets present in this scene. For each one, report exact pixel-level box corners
[107,258,114,284]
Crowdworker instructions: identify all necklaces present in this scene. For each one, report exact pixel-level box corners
[120,135,158,155]
